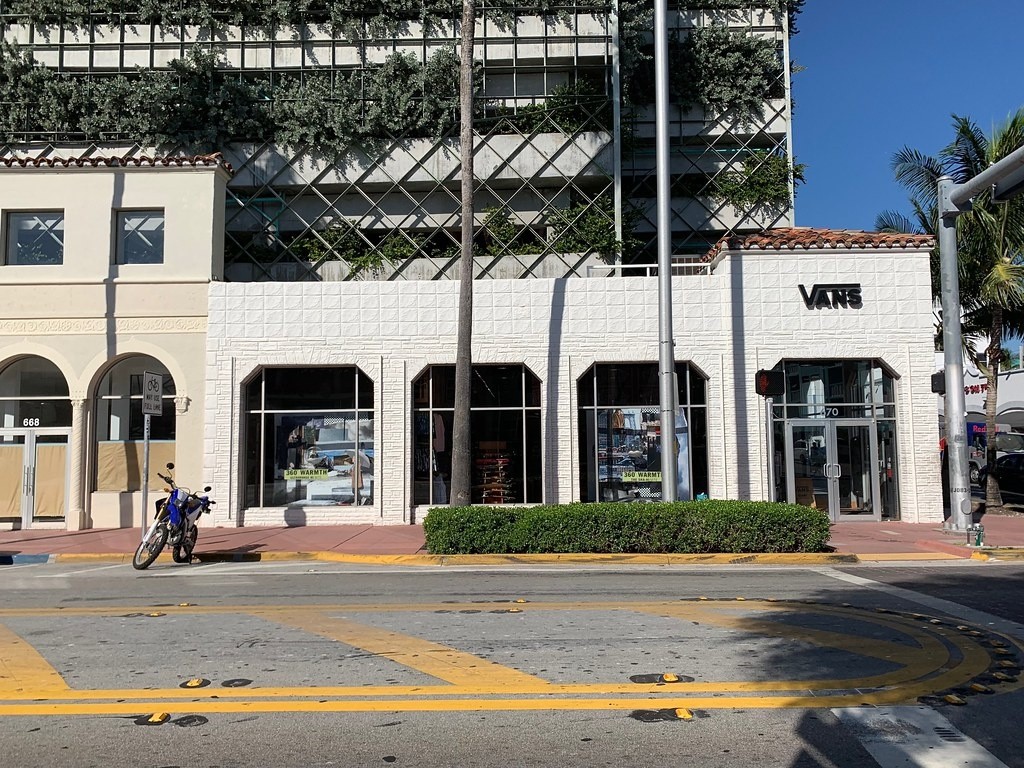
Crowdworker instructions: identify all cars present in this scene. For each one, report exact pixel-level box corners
[979,453,1024,505]
[793,440,807,464]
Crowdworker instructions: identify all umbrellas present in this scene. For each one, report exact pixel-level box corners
[346,449,370,471]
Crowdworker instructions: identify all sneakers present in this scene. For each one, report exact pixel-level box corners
[360,497,367,505]
[350,501,359,506]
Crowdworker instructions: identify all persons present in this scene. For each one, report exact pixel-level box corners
[351,456,367,506]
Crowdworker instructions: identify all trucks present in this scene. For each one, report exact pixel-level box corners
[939,421,1024,484]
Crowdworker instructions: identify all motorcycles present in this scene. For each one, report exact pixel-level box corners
[132,463,217,570]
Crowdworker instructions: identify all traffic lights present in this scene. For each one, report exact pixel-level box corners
[755,369,785,395]
